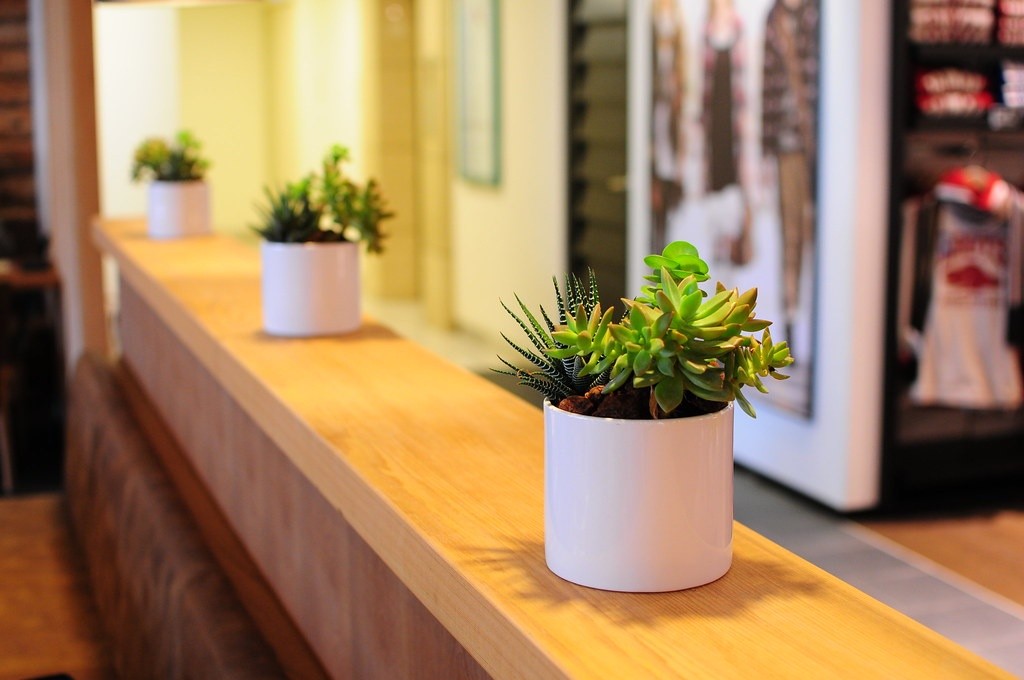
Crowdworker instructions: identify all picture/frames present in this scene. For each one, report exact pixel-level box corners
[623,1,889,513]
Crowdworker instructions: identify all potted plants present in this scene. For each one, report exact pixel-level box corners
[489,239,792,594]
[129,131,210,237]
[251,146,394,339]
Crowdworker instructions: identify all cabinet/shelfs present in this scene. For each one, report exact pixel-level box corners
[877,0,1024,520]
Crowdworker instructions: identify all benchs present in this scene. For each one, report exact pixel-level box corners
[0,350,291,679]
